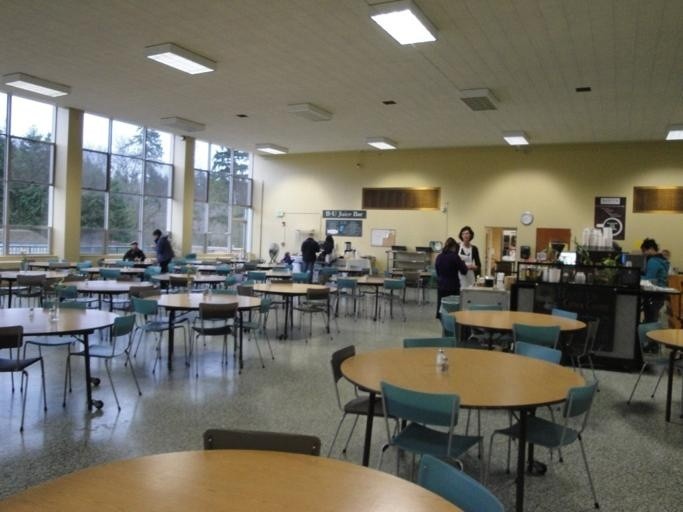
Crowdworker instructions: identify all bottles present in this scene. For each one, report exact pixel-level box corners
[49,308,55,321]
[53,298,60,318]
[435,348,448,373]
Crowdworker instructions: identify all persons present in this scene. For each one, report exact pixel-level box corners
[434,237,467,318]
[122,240,146,262]
[660,249,673,274]
[317,233,333,264]
[152,229,176,289]
[457,226,481,287]
[300,231,320,272]
[641,238,671,355]
[282,252,295,265]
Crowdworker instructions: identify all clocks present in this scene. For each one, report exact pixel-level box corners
[520,213,534,224]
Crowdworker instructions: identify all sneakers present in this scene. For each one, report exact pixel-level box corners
[643,344,659,353]
